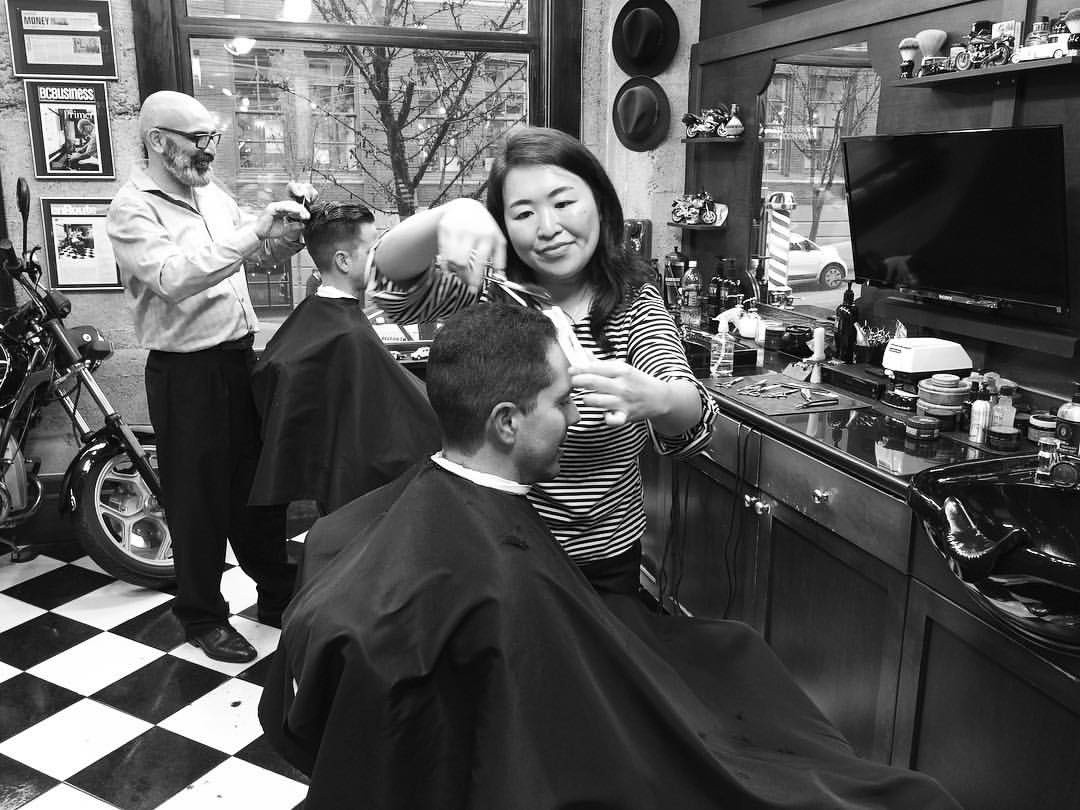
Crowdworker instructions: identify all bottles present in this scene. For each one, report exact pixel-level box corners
[663,246,690,308]
[960,376,1018,444]
[679,259,702,326]
[710,313,736,377]
[1055,394,1079,455]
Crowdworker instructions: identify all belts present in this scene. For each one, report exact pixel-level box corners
[211,331,255,351]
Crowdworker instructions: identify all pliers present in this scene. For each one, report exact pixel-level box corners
[794,398,839,409]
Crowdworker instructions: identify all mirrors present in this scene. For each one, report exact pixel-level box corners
[750,38,882,318]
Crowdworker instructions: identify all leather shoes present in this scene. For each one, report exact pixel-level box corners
[184,620,257,664]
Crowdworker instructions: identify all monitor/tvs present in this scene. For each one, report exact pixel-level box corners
[833,122,1073,323]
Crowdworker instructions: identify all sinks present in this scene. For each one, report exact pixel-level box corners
[906,453,1080,660]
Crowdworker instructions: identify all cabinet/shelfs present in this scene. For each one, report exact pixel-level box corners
[634,394,1080,809]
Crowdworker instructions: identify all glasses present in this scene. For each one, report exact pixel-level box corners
[154,126,223,150]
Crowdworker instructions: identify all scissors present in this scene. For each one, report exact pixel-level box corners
[715,376,800,400]
[481,268,550,308]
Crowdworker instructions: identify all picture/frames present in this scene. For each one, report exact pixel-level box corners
[3,0,118,83]
[23,78,116,182]
[41,195,124,295]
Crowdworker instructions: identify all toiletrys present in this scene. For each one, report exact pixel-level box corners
[830,279,870,365]
[750,253,772,304]
[659,245,745,331]
[904,371,1080,459]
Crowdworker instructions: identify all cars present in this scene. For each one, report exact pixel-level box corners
[785,231,845,290]
[1012,34,1070,62]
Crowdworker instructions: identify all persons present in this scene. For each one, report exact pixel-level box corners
[362,126,720,598]
[103,91,321,663]
[300,197,439,499]
[395,299,926,810]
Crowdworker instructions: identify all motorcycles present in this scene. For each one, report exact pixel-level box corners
[1,175,177,593]
[956,31,1012,70]
